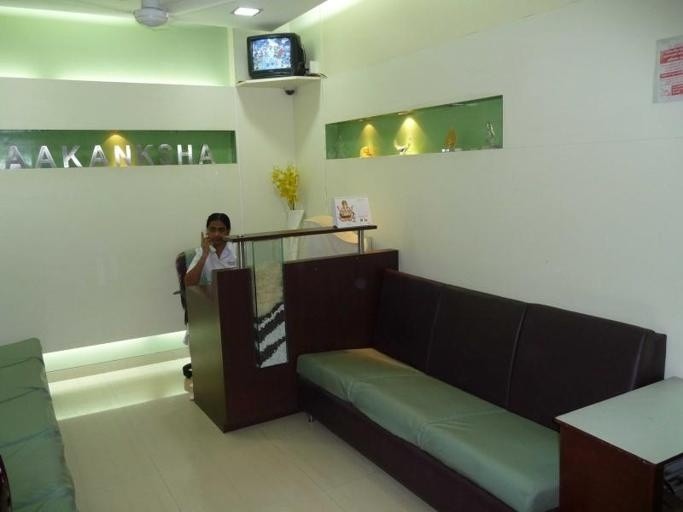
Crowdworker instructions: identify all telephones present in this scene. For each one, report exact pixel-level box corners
[203,232,217,254]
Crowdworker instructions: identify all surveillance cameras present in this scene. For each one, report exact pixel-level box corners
[284,88,295,95]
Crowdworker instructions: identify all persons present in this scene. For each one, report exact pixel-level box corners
[184,213,237,287]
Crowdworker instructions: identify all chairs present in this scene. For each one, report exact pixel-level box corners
[174,250,193,380]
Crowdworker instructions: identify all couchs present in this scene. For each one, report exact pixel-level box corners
[293,269,659,509]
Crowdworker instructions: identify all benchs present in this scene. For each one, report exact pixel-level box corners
[0,337,79,510]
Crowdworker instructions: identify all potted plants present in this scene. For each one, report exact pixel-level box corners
[270,160,303,229]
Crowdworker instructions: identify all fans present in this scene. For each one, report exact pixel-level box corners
[120,1,231,29]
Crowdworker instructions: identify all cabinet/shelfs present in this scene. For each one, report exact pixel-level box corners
[553,374,683,509]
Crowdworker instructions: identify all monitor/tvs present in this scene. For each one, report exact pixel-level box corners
[246,33,306,80]
